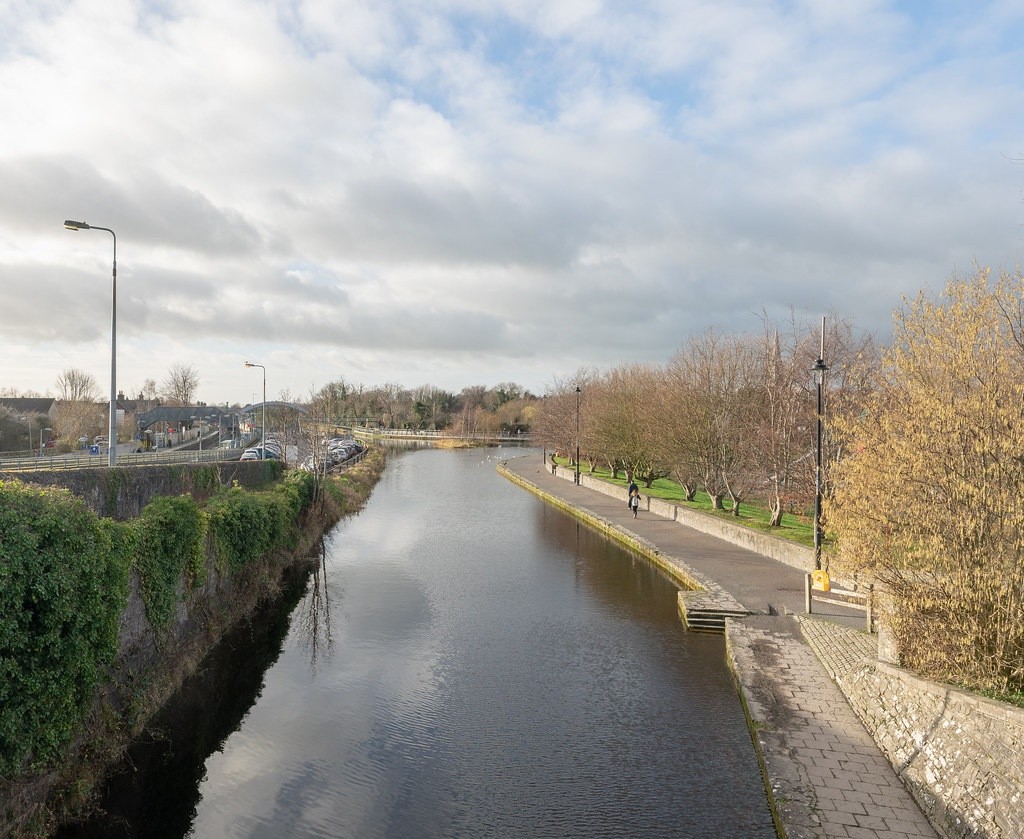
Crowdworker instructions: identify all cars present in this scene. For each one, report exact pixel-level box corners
[238,436,365,474]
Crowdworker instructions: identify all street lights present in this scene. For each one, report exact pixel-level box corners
[63,220,119,468]
[573,385,582,487]
[190,415,211,450]
[39,428,52,456]
[542,395,549,465]
[225,411,259,447]
[809,354,830,572]
[210,413,230,450]
[244,362,268,462]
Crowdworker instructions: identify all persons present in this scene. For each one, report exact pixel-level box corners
[627,480,638,510]
[502,426,523,437]
[629,489,641,519]
[133,428,200,453]
[42,441,45,449]
